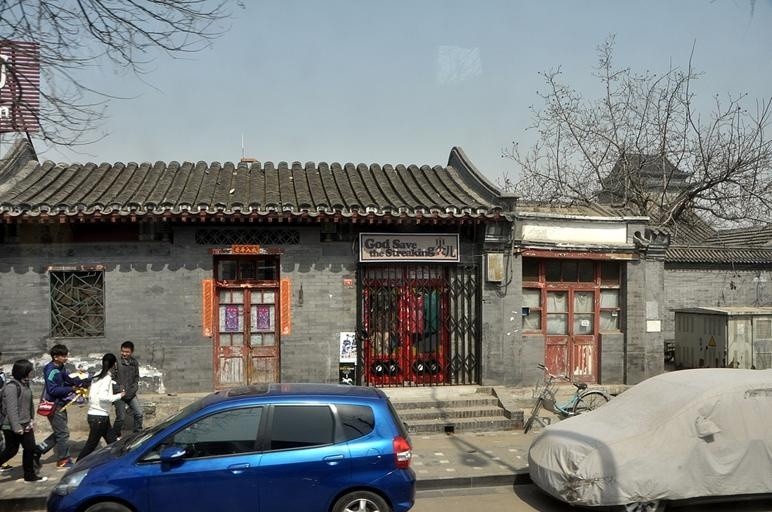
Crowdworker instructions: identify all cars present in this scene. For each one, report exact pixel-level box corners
[575,493,772,512]
[46,383,416,512]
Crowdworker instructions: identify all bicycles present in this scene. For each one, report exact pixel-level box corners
[523,363,611,435]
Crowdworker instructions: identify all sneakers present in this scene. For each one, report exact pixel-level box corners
[32,444,43,467]
[55,456,77,469]
[0,463,15,472]
[23,474,49,484]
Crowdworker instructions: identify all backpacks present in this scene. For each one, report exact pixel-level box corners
[0,380,21,430]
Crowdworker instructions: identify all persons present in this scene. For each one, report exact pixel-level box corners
[33,343,91,468]
[1,358,45,481]
[112,340,144,440]
[76,352,126,464]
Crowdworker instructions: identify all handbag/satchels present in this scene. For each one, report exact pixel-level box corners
[37,399,58,418]
